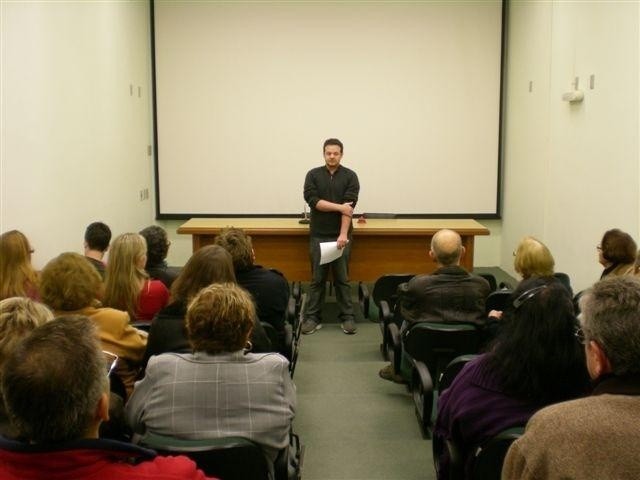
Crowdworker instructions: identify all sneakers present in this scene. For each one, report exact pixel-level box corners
[378,363,404,385]
[299,317,324,335]
[339,318,358,335]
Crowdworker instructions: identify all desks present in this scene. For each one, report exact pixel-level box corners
[176,216,490,296]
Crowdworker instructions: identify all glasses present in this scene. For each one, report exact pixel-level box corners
[99,347,120,380]
[29,246,35,257]
[513,249,517,256]
[573,327,592,345]
[596,244,604,252]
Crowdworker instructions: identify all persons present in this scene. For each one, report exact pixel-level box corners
[1,220,290,478]
[301,138,361,333]
[380,227,638,380]
[435,274,586,455]
[500,276,640,480]
